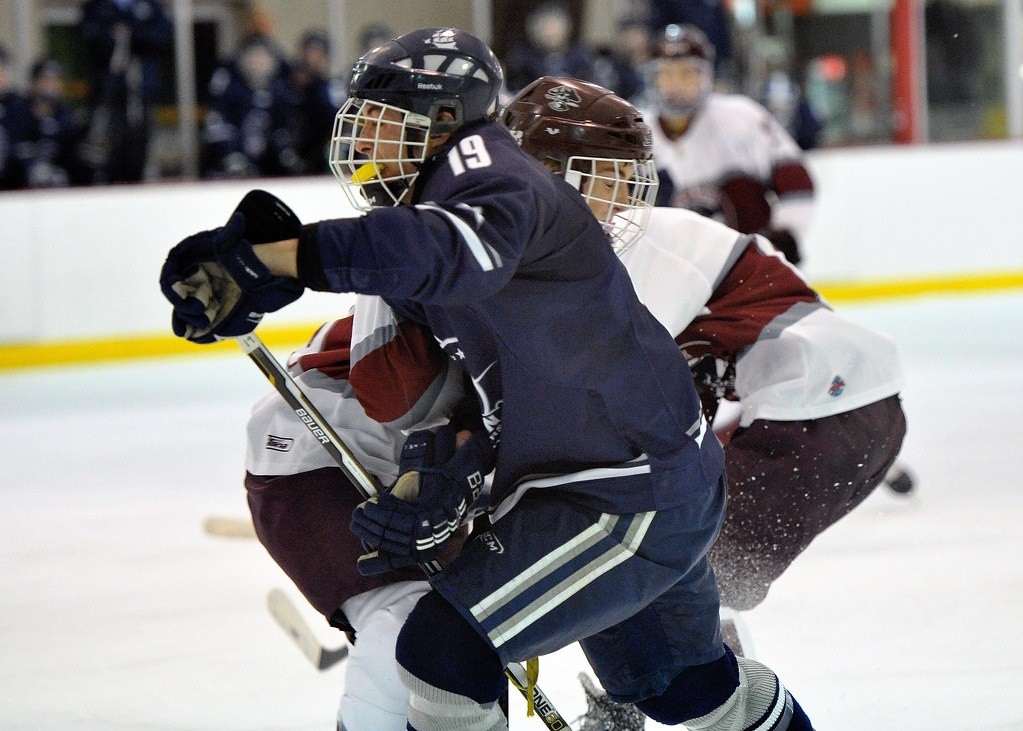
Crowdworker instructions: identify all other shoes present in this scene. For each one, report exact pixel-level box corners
[569,672,646,731]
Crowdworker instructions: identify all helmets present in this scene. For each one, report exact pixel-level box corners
[329,27,503,212]
[489,75,660,258]
[646,21,716,63]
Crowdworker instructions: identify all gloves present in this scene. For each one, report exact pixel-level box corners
[159,189,305,345]
[347,430,497,577]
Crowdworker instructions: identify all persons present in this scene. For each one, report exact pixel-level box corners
[159,28,818,730]
[249,24,910,731]
[1,1,250,191]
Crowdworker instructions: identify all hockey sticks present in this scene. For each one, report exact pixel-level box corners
[236,328,580,731]
[264,587,349,672]
